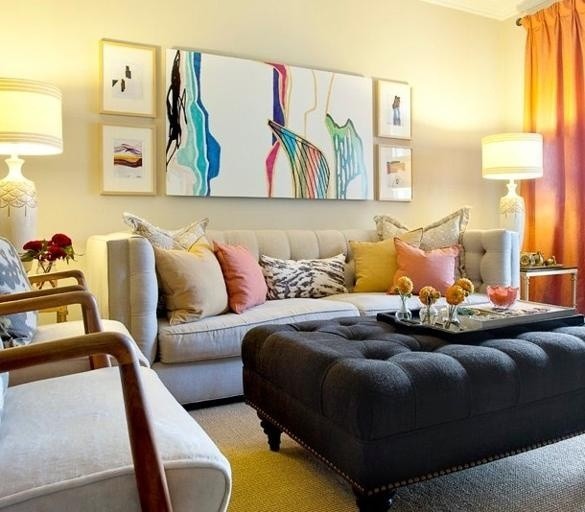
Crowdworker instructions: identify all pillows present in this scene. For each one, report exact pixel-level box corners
[372,207,472,281]
[152,234,228,326]
[120,211,209,249]
[260,253,346,300]
[389,235,461,296]
[211,239,268,314]
[346,229,425,291]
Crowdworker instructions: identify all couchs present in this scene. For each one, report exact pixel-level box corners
[85,228,519,407]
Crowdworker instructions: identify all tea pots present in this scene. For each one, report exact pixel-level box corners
[532,251,544,266]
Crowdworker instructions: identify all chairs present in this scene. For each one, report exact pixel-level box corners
[0,233,150,385]
[0,289,235,512]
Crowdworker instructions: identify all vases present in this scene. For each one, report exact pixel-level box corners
[396,296,460,332]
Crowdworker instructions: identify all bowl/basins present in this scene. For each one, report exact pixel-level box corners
[486,284,520,308]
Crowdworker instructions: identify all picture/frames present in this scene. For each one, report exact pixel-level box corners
[97,122,158,196]
[375,143,413,201]
[98,37,157,116]
[376,80,412,139]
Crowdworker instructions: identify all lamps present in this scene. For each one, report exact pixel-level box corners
[480,132,543,251]
[0,77,63,273]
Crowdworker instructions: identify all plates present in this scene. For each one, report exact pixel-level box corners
[519,264,565,271]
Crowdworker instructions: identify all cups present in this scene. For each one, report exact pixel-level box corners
[519,252,536,267]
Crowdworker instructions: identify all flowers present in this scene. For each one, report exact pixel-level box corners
[419,286,440,322]
[396,276,413,318]
[453,278,474,295]
[18,234,84,289]
[444,285,465,329]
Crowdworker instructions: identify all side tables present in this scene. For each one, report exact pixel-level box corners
[520,263,578,307]
